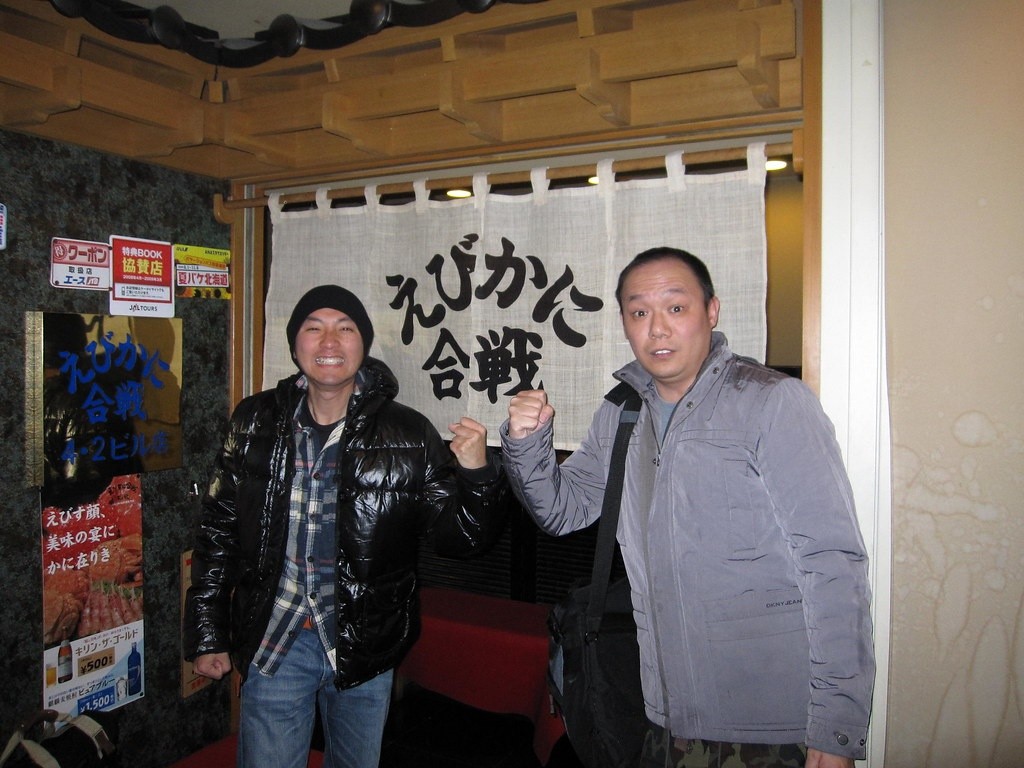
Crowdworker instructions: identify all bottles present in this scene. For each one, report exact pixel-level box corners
[128,642,141,696]
[57,640,73,683]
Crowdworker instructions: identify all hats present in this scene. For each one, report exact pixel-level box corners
[285,283,374,373]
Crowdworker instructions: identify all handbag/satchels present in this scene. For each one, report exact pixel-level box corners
[0,708,120,768]
[543,577,648,768]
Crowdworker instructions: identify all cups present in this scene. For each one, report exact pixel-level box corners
[45,663,57,689]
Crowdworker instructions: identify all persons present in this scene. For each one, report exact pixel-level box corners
[186,284,492,767]
[500,246,877,767]
